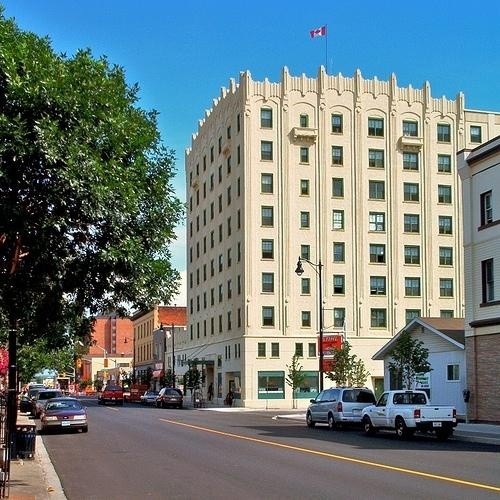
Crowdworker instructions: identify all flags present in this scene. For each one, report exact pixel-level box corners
[310,25,326,38]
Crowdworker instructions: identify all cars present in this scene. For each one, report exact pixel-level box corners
[96,384,161,407]
[19,381,88,434]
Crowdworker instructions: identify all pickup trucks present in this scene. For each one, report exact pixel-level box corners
[360,390,460,439]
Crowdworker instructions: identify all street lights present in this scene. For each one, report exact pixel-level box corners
[159,321,176,389]
[123,335,136,383]
[295,255,325,392]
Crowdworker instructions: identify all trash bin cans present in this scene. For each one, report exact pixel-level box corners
[15,425,36,460]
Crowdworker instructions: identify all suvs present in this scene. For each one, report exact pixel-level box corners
[157,387,185,408]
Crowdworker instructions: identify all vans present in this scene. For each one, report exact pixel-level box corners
[305,386,377,430]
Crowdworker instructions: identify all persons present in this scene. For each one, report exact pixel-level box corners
[208,382,214,401]
[226,391,234,407]
